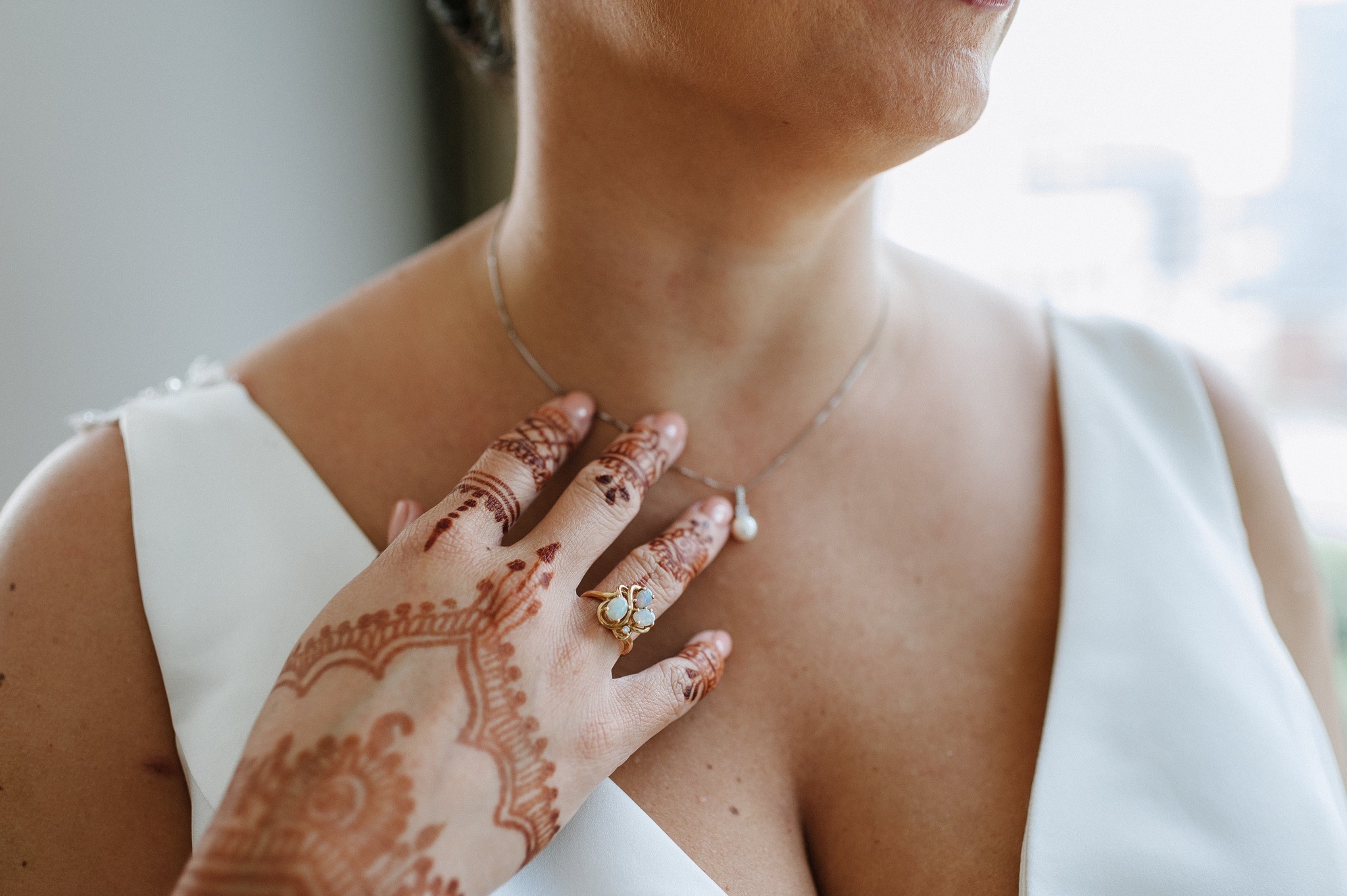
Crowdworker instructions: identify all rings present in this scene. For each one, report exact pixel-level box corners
[581,584,657,654]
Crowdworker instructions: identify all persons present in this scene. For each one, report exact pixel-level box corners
[0,8,1344,893]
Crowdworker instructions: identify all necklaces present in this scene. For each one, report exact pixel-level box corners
[490,193,896,542]
